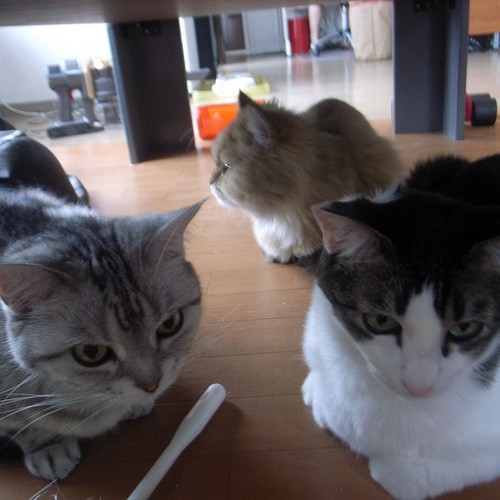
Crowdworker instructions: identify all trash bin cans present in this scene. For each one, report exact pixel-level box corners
[289,17,310,54]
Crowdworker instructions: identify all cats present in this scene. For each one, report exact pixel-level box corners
[300,151,500,500]
[0,184,211,482]
[209,88,404,263]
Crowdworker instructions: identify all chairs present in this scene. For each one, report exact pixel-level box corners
[311,3,353,59]
